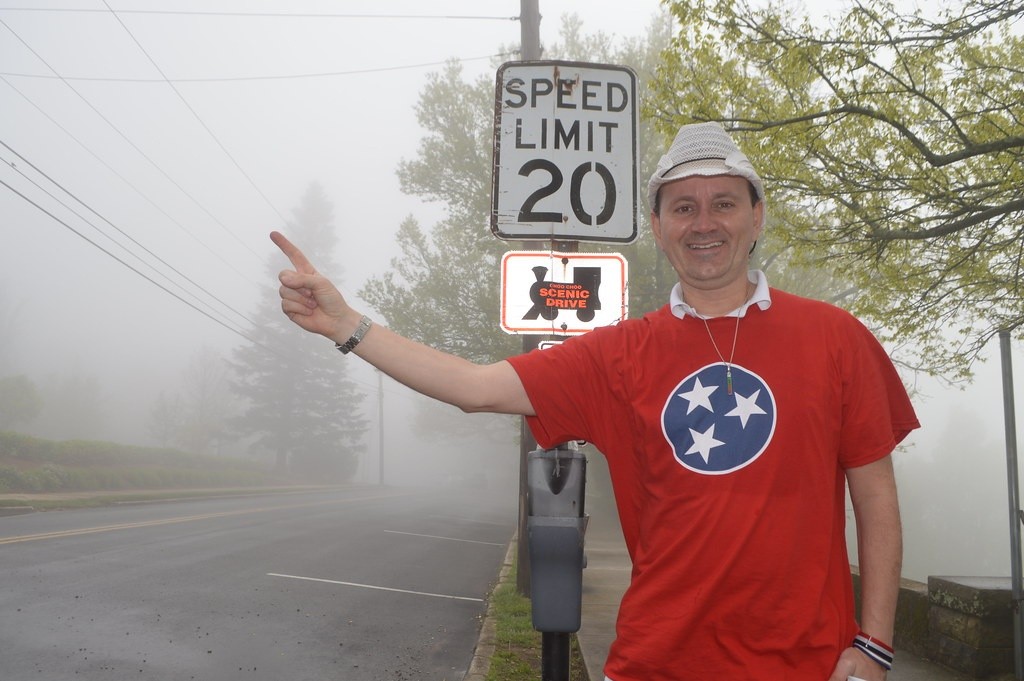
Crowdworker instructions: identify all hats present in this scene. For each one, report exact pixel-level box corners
[647,121,766,233]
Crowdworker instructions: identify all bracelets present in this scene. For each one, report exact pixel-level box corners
[334,315,372,355]
[849,630,895,671]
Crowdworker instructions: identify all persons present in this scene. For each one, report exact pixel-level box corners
[270,121,924,681]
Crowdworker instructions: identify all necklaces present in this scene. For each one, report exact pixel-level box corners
[693,287,749,395]
